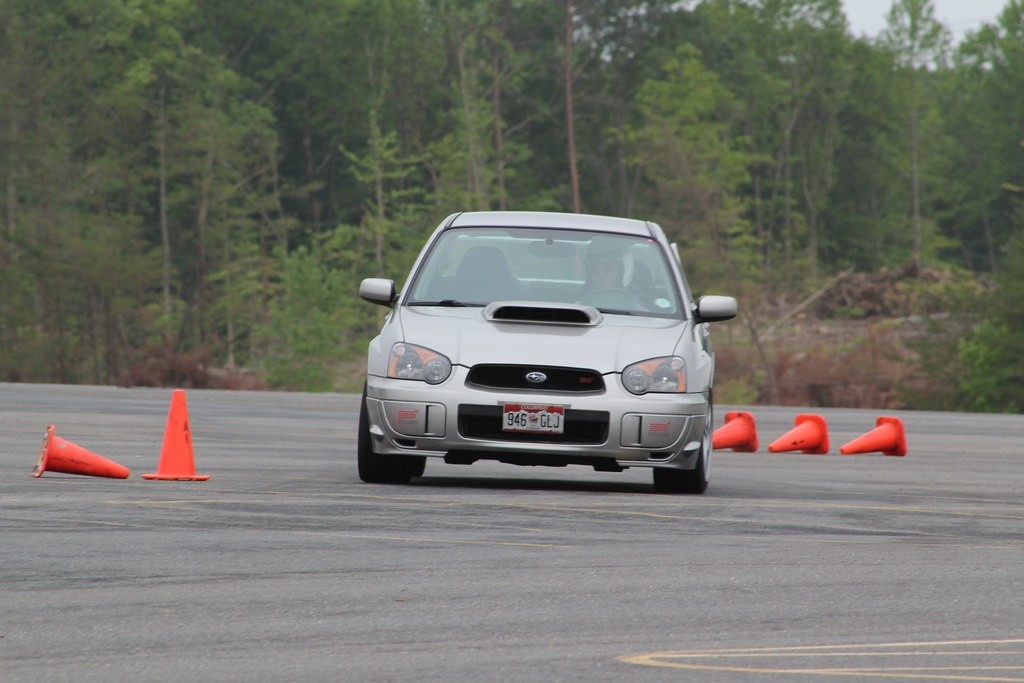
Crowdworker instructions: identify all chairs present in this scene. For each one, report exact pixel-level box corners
[454,245,521,303]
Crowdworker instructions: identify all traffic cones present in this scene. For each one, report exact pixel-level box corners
[142,388,212,482]
[769,413,831,456]
[33,424,130,479]
[840,414,907,457]
[710,409,759,452]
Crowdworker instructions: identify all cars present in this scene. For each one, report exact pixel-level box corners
[357,209,741,494]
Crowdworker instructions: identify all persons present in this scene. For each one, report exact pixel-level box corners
[576,249,650,312]
